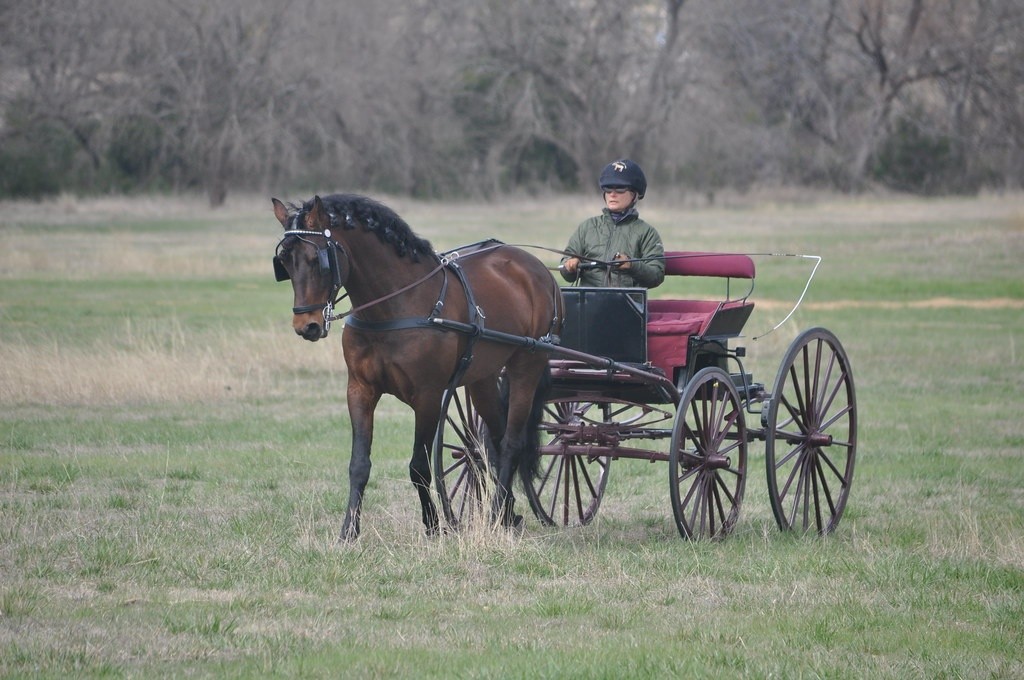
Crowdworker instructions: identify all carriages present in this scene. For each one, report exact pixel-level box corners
[269,190,860,546]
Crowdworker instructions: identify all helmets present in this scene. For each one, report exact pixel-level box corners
[599,158,647,200]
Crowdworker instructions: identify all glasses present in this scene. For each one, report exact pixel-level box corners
[602,185,636,195]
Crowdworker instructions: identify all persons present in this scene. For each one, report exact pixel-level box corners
[559,159,667,305]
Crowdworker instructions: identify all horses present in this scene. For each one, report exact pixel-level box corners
[270,194,565,542]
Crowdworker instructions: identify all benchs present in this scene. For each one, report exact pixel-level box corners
[648,247,756,387]
